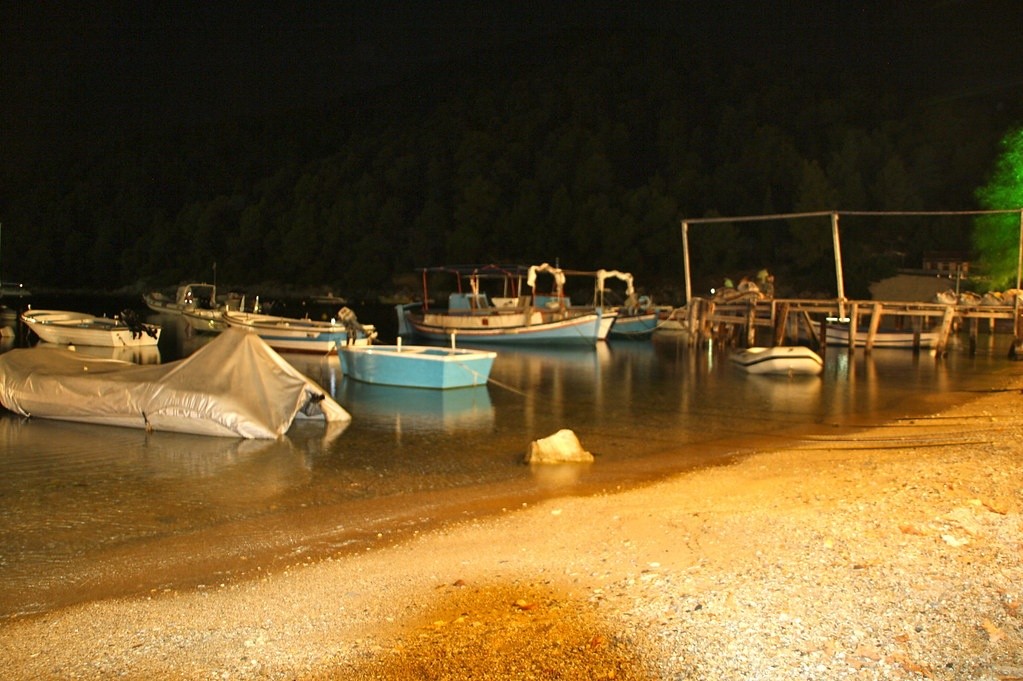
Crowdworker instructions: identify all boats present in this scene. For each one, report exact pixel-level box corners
[143,283,377,354]
[0,324,350,440]
[334,334,497,389]
[398,265,660,342]
[730,346,825,375]
[784,316,938,350]
[0,303,162,346]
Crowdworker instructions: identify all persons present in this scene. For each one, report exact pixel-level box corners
[723,276,732,287]
[756,267,768,295]
[626,290,651,317]
